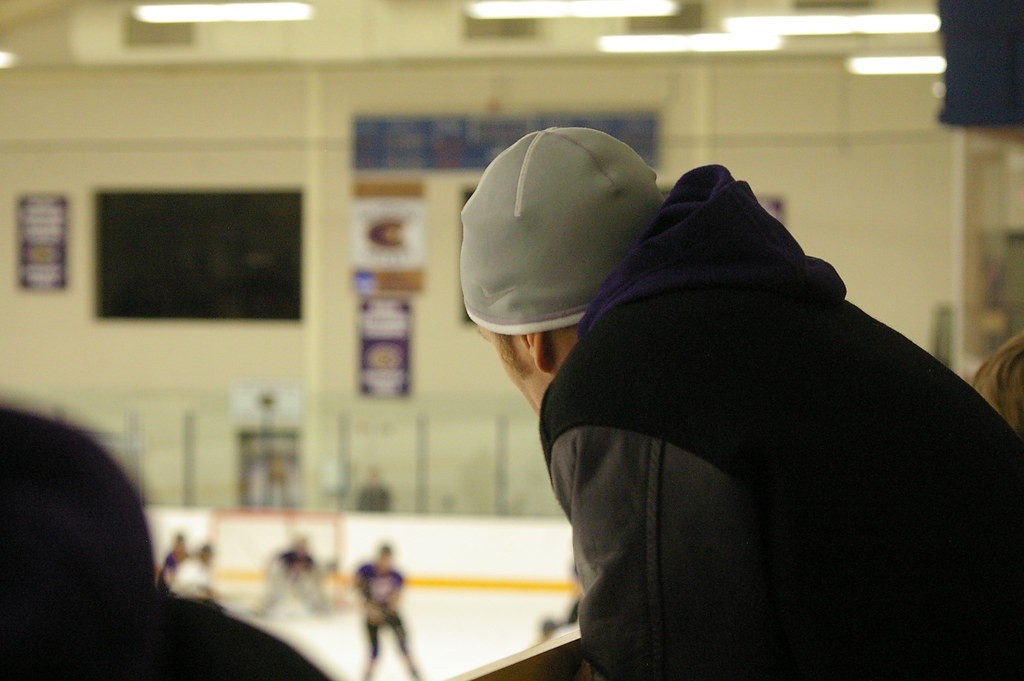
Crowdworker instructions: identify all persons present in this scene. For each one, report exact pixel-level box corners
[973,328,1022,438]
[1,405,426,681]
[458,126,1024,681]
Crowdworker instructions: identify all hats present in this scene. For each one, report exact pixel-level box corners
[461,127,666,334]
